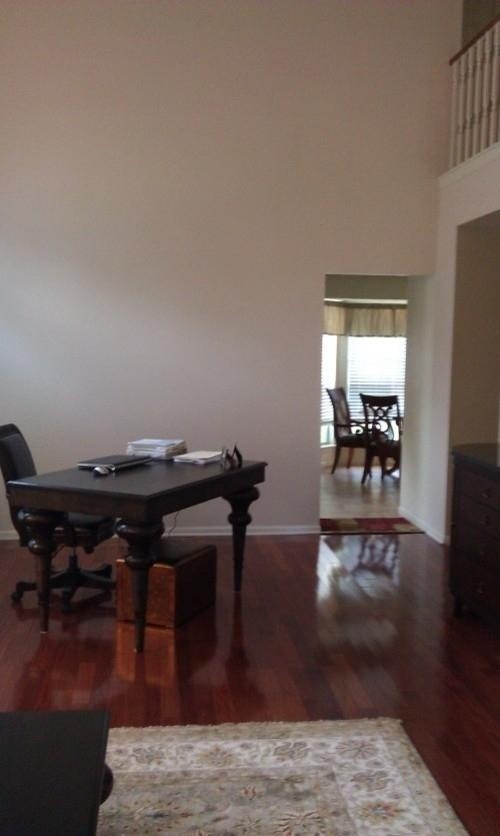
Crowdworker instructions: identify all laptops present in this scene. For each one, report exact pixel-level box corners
[77,454,154,471]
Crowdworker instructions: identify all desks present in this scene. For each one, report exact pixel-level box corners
[7,454,268,651]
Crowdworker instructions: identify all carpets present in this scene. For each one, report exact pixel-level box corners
[96,715,470,836]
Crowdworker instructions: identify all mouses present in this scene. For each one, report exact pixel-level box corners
[92,466,110,475]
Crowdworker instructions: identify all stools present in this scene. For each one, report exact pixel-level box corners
[116,536,217,628]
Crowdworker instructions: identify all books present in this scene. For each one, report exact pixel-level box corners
[169,447,225,465]
[123,436,189,464]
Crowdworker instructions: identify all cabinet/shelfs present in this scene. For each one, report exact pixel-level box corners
[449,464,500,629]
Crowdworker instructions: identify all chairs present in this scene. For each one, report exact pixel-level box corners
[326,387,403,484]
[0,711,113,836]
[0,423,118,611]
